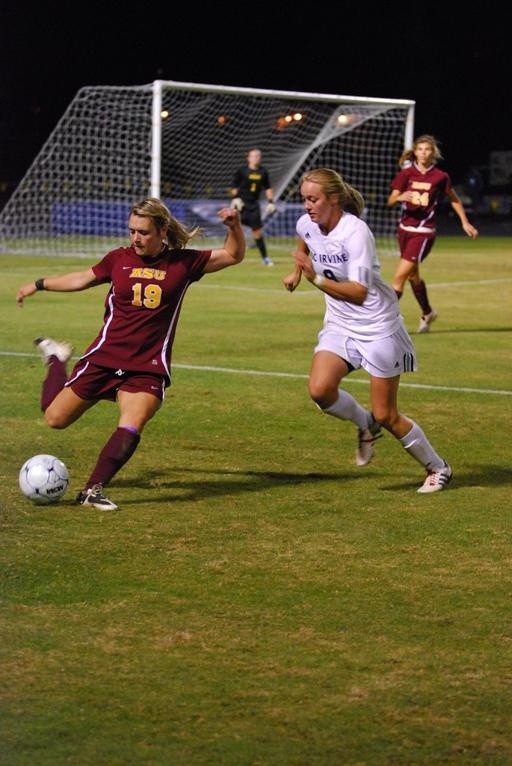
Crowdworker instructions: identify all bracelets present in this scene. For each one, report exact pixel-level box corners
[311,273,324,286]
[34,277,45,290]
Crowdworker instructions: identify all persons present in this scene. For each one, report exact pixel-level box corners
[384,132,480,333]
[280,166,455,496]
[15,195,247,513]
[223,146,277,268]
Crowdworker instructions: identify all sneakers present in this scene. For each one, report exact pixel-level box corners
[417,310,439,334]
[414,456,452,494]
[355,410,382,469]
[262,255,274,268]
[33,336,75,363]
[74,484,119,511]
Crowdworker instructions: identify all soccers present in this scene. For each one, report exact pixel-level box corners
[18,454,68,505]
